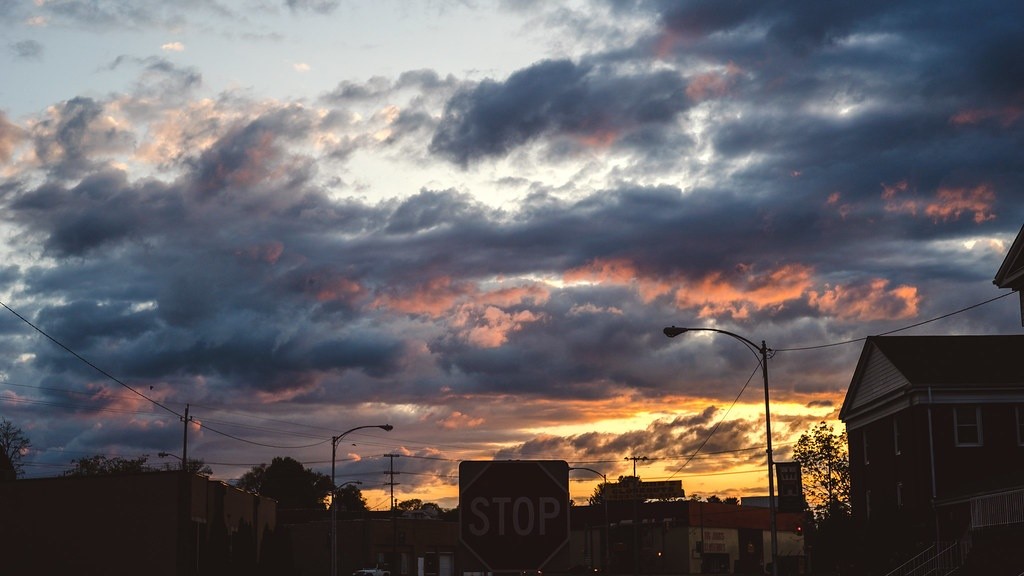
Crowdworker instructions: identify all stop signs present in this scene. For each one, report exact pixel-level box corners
[458,459,570,573]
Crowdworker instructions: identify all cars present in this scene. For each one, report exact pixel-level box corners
[352,564,391,576]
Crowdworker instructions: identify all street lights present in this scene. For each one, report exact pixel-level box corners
[567,466,609,576]
[158,452,189,576]
[664,325,780,576]
[330,424,394,576]
